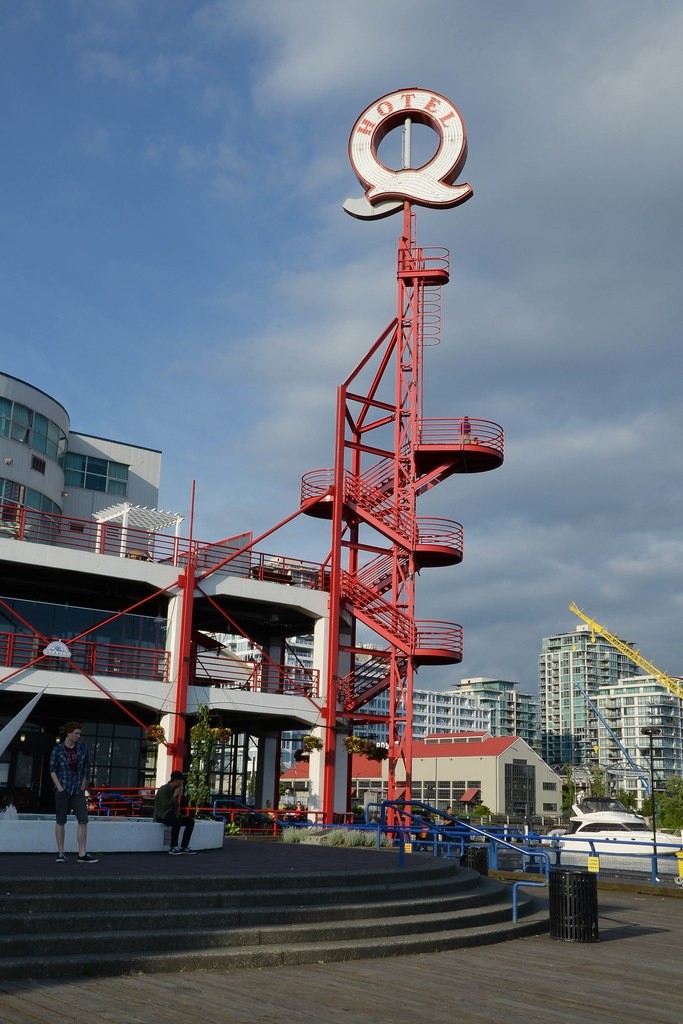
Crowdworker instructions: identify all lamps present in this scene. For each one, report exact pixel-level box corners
[4,456,13,464]
[61,491,68,497]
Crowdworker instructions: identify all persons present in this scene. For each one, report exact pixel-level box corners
[265,800,274,819]
[49,722,99,864]
[293,801,303,819]
[84,783,107,809]
[444,806,459,826]
[153,771,197,854]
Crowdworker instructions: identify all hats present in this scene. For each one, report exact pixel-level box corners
[171,771,187,780]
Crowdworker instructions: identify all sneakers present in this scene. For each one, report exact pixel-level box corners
[168,846,186,855]
[56,852,66,863]
[179,847,197,854]
[77,852,99,863]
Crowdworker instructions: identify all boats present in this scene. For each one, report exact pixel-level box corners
[538,797,683,857]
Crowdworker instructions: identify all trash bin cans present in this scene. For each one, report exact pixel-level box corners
[548,868,602,943]
[460,845,489,877]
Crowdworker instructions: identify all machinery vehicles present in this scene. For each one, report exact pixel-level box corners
[567,599,683,698]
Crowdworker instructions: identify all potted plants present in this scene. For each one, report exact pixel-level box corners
[344,735,389,763]
[145,725,167,744]
[303,736,324,752]
[212,728,232,743]
